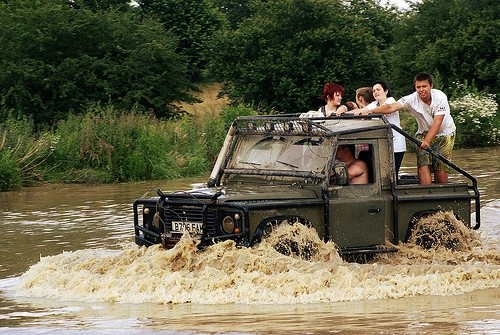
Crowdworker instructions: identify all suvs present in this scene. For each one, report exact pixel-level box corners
[132,111,481,266]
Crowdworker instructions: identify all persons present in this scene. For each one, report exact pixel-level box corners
[346,87,375,109]
[354,72,456,185]
[336,145,369,185]
[336,80,407,185]
[307,83,348,117]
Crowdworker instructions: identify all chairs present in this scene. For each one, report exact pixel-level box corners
[358,150,374,184]
